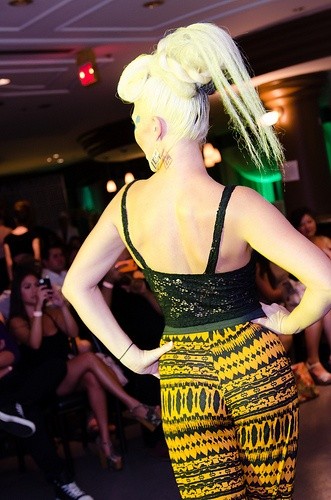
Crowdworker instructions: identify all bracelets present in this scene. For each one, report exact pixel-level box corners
[118,341,135,361]
[32,311,43,317]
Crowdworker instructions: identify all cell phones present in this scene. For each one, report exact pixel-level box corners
[39,278,52,298]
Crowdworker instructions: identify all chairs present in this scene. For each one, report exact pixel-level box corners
[18,395,128,477]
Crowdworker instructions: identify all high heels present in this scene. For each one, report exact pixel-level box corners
[95,438,123,470]
[306,360,331,385]
[136,402,162,432]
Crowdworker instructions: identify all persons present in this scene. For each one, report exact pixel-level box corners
[61,23,331,500]
[0,199,331,500]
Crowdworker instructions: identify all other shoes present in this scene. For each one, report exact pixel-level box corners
[76,416,116,436]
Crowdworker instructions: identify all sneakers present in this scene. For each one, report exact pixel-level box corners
[54,482,95,500]
[0,402,36,437]
